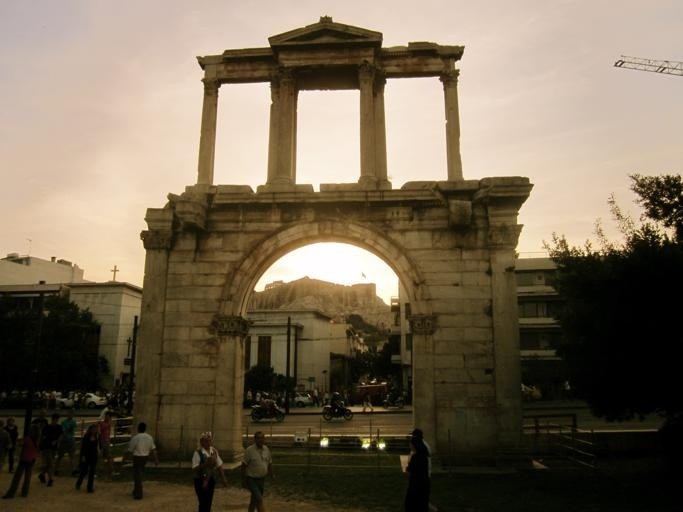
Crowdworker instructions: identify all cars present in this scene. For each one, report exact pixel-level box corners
[57,391,108,409]
[282,392,313,407]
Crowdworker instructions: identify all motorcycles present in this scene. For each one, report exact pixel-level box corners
[322,401,354,422]
[383,393,406,409]
[251,401,284,422]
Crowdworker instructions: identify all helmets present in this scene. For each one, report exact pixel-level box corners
[263,392,271,399]
[332,391,340,398]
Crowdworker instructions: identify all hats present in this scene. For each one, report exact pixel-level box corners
[410,427,424,438]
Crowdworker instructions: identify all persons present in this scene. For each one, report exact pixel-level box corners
[240,430,277,512]
[0,424,40,499]
[259,392,274,415]
[389,387,398,402]
[361,386,374,413]
[406,428,432,511]
[246,386,330,408]
[111,378,120,408]
[401,435,432,511]
[0,408,123,494]
[330,391,343,417]
[190,430,230,511]
[124,422,159,501]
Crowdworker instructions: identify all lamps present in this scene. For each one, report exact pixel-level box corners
[361,440,370,448]
[376,441,386,450]
[320,436,329,447]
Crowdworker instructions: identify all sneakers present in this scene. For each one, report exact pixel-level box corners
[37,472,47,483]
[46,478,54,488]
